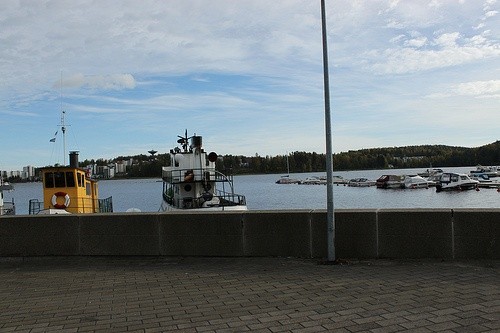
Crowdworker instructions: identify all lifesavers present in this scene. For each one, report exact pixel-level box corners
[52,192,70,209]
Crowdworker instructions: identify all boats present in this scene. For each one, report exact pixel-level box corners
[156,128,248,211]
[400,175,428,190]
[436,172,480,191]
[417,165,500,188]
[28,149,114,215]
[376,174,402,189]
[274,173,376,190]
[0,172,17,214]
